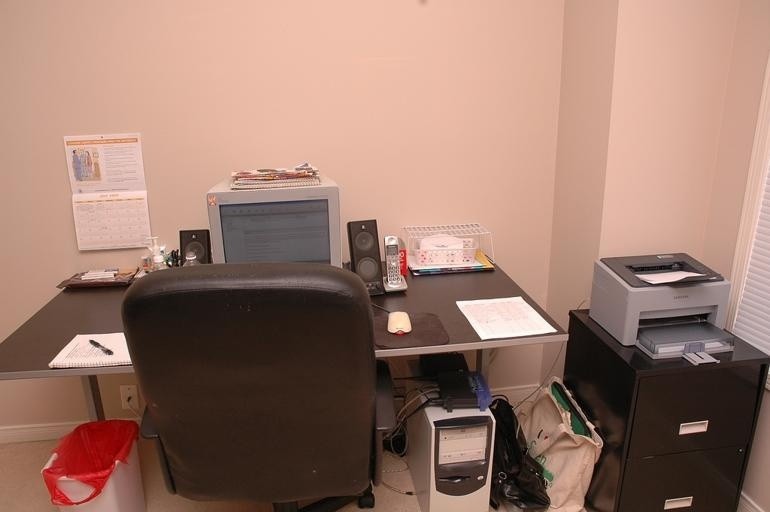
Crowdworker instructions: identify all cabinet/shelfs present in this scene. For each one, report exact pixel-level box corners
[561,307,770,512]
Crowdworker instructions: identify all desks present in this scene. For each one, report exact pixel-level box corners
[0,255,567,420]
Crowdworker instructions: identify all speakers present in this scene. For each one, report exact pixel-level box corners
[347,219,386,296]
[180,229,209,265]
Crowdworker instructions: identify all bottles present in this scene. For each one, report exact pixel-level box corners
[142,257,149,273]
[183,252,201,267]
[151,255,169,272]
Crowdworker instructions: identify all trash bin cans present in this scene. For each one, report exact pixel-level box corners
[40,419,146,512]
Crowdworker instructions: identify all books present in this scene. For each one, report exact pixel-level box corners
[230,160,321,190]
[48,332,133,368]
[81,267,119,280]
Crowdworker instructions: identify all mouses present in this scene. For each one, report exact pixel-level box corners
[387,311,413,335]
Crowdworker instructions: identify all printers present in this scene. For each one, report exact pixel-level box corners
[588,252,734,367]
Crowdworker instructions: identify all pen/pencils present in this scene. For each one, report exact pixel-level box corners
[90,340,113,355]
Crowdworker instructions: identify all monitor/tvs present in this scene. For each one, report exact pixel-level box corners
[208,163,342,267]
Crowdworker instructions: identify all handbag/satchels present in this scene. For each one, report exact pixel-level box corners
[489,395,550,512]
[514,376,603,512]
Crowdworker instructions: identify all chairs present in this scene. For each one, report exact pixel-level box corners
[121,261,384,512]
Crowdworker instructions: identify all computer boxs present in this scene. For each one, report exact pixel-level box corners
[406,359,497,512]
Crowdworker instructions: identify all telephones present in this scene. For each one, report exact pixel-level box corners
[383,236,408,292]
